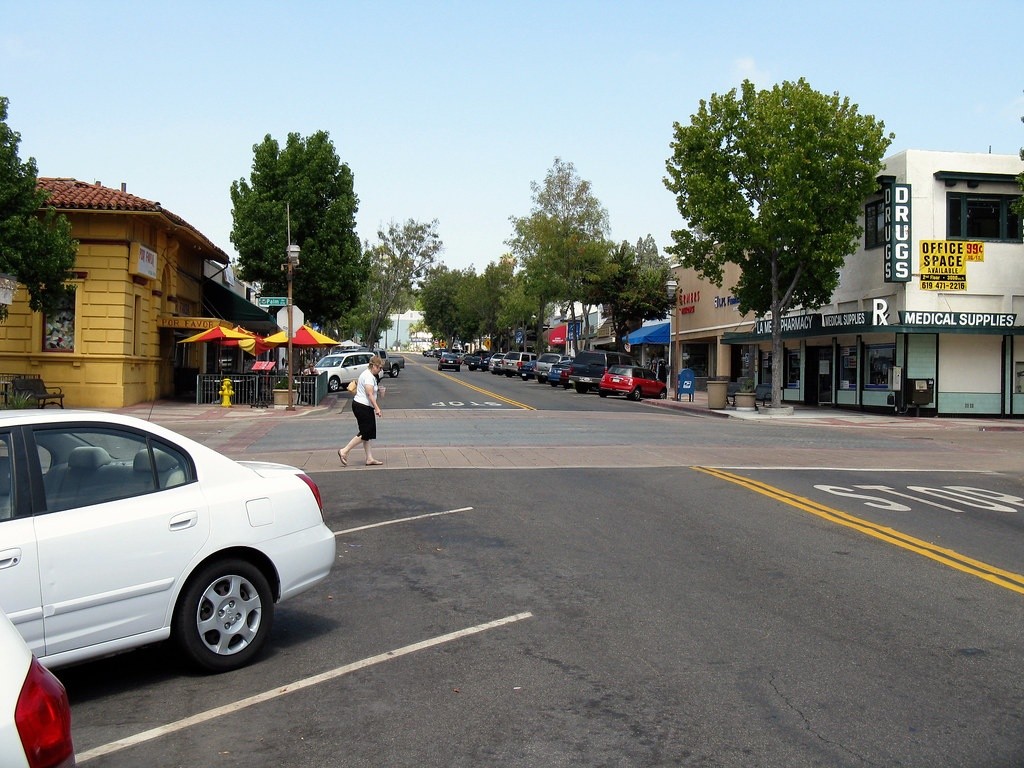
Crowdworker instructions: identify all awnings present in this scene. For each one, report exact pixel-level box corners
[157,315,233,330]
[621,321,670,346]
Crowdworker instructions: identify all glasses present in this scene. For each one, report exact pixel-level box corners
[309,366,314,367]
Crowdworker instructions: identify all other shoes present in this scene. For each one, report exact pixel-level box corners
[338,449,347,465]
[366,460,383,465]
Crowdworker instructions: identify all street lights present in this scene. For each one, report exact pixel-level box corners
[281,201,301,411]
[656,290,679,401]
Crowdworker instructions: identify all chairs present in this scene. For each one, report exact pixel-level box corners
[677,368,695,402]
[726,383,742,405]
[13,379,65,407]
[753,384,773,406]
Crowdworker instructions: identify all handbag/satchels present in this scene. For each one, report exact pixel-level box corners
[346,380,358,394]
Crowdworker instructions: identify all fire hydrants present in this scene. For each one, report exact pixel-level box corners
[219,378,235,407]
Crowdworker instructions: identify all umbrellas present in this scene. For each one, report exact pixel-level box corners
[262,323,341,373]
[338,339,361,346]
[175,323,278,372]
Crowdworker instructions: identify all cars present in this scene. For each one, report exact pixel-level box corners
[559,369,575,389]
[547,360,573,387]
[0,606,74,754]
[333,349,358,354]
[533,353,575,383]
[488,352,505,376]
[302,352,384,393]
[459,354,472,366]
[422,348,469,358]
[437,354,460,372]
[516,359,536,381]
[0,410,337,672]
[598,364,667,401]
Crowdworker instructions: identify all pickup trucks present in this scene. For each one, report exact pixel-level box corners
[356,348,404,378]
[464,351,495,372]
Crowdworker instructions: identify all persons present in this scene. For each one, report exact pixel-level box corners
[304,363,319,376]
[338,356,385,466]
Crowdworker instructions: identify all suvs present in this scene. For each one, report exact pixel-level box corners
[568,349,638,394]
[500,351,537,377]
